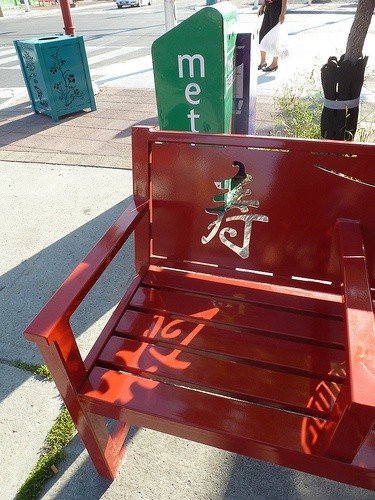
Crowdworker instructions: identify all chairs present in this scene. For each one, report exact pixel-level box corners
[23,124,375,491]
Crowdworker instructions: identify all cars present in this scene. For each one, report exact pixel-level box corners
[116,0,152,9]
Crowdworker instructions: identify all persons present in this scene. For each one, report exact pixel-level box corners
[255,0,286,72]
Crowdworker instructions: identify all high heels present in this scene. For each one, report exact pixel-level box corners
[259,62,278,72]
